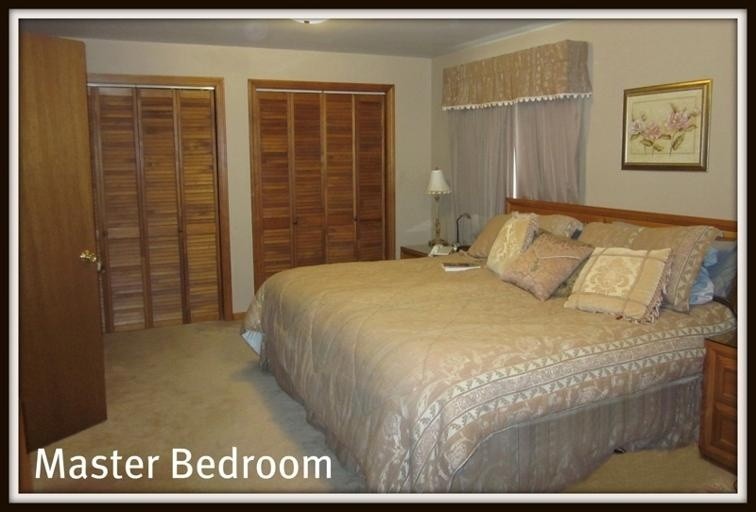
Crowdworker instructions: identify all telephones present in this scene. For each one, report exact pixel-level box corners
[428,243,452,257]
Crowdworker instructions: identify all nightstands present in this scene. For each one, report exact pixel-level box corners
[400,245,472,259]
[699,329,737,475]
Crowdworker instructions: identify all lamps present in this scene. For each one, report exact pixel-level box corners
[422,167,453,247]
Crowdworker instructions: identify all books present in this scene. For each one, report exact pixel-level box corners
[440,261,481,271]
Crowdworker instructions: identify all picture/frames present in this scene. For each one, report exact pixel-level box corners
[622,78,712,171]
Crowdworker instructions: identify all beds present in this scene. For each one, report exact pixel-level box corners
[260,197,738,492]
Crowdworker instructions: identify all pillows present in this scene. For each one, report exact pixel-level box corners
[467,210,737,325]
